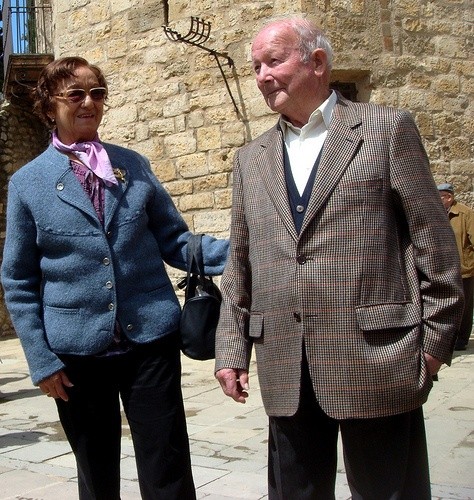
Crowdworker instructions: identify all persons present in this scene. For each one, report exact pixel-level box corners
[215,15,466,500]
[437,183,474,353]
[0,57,232,500]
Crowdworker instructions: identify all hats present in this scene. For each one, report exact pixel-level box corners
[436,184,454,191]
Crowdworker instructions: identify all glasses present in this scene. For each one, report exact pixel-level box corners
[52,87,108,103]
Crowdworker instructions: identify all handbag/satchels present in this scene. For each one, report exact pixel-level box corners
[176,233,223,362]
